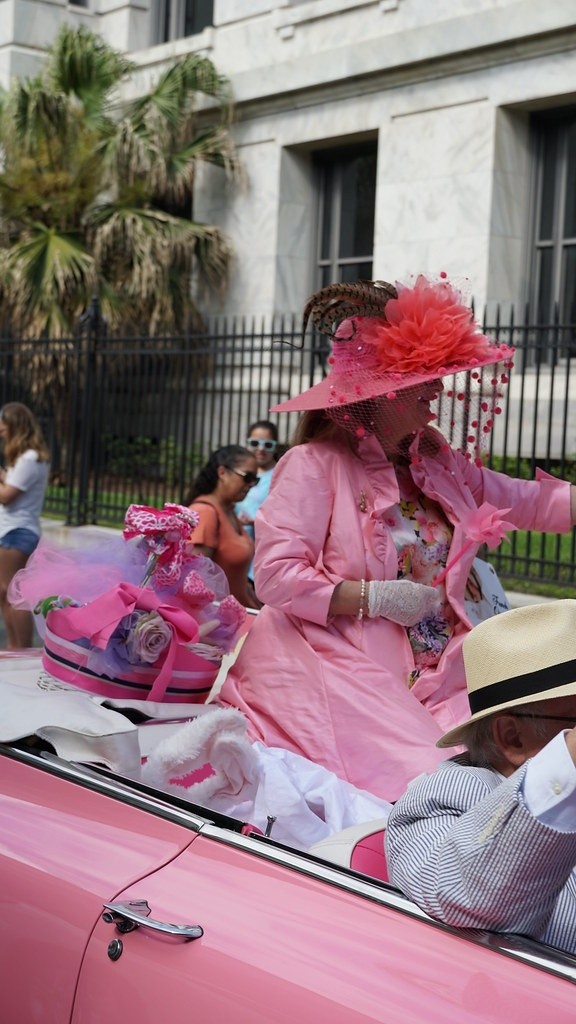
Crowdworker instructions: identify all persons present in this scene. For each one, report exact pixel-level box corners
[1,402,46,648]
[216,282,576,805]
[384,597,576,956]
[233,419,292,590]
[180,441,261,609]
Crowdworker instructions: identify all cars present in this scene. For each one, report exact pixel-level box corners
[0,646,576,1024]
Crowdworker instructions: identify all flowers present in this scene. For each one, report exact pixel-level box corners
[87,610,173,681]
[367,275,493,376]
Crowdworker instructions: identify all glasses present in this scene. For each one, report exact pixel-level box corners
[247,437,277,451]
[228,466,259,486]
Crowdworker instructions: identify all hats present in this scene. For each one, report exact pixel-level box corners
[433,599,576,749]
[268,272,515,483]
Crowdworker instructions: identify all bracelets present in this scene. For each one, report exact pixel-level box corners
[355,577,366,621]
